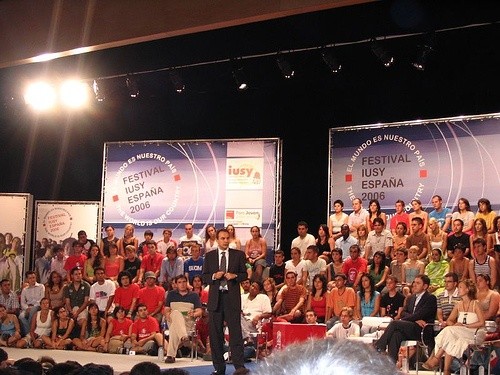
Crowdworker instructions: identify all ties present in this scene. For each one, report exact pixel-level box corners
[219,252,227,288]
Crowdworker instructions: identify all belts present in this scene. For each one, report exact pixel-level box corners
[220,289,229,294]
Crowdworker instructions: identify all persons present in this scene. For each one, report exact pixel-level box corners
[390,200,410,236]
[201,229,248,375]
[329,200,348,241]
[429,195,453,233]
[410,200,429,234]
[0,217,500,375]
[366,199,387,233]
[451,198,474,233]
[473,198,497,233]
[348,198,371,240]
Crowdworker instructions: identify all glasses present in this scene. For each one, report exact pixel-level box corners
[286,276,294,279]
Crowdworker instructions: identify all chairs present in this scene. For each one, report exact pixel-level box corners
[347,316,500,374]
[164,316,268,364]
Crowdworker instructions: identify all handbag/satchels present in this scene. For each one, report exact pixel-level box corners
[464,343,497,365]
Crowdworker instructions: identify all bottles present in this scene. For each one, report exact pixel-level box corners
[402,356,408,374]
[479,363,484,375]
[460,362,466,375]
[158,346,164,362]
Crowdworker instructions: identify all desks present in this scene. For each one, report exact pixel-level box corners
[272,321,330,352]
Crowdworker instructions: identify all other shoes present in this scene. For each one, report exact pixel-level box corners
[117,347,124,354]
[374,345,384,352]
[182,336,191,347]
[210,369,225,375]
[165,356,175,363]
[422,356,442,370]
[95,344,103,351]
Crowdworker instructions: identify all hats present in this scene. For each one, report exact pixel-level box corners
[145,271,156,279]
[286,270,297,275]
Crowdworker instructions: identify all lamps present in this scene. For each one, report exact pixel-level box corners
[222,62,251,93]
[408,40,441,72]
[365,39,397,69]
[90,79,110,105]
[315,46,346,73]
[124,76,142,100]
[272,55,298,82]
[170,75,188,96]
